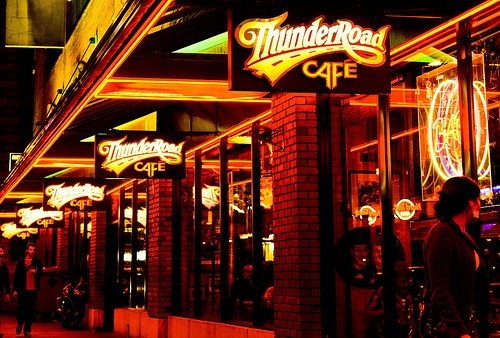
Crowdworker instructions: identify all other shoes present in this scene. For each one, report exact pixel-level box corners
[16,324,22,334]
[25,332,31,338]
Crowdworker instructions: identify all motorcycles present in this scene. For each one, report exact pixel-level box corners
[56,277,85,329]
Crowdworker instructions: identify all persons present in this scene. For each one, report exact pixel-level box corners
[230,261,254,315]
[0,246,11,306]
[12,244,44,338]
[348,240,378,285]
[422,177,490,338]
